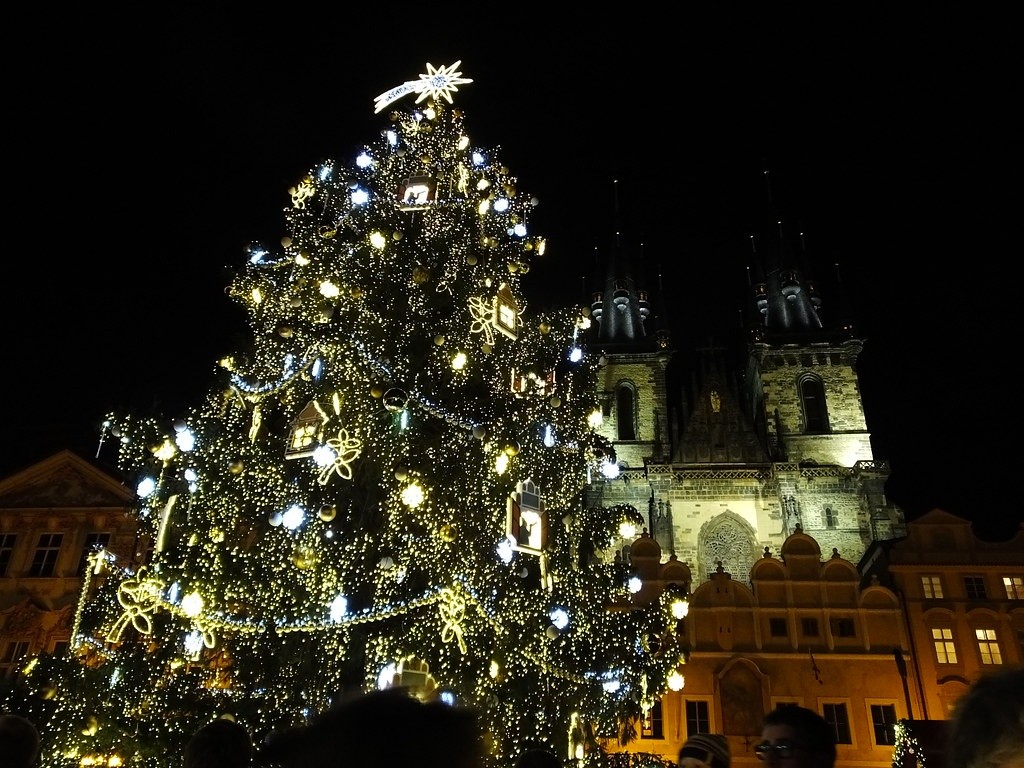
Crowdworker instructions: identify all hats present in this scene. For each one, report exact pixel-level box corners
[679,733,730,768]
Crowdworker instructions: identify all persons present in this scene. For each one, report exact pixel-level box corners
[0,660,1023,768]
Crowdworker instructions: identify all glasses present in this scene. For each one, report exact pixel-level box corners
[754,743,795,760]
